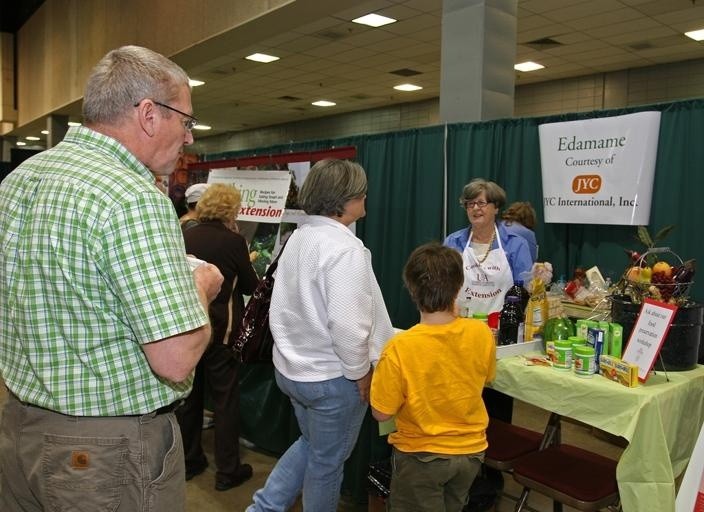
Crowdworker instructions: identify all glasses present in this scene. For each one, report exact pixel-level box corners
[132,98,201,130]
[466,200,494,208]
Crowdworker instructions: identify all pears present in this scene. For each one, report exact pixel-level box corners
[637,266,652,283]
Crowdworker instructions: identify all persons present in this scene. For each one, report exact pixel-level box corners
[175,181,258,491]
[500,198,543,291]
[177,180,210,225]
[0,42,225,510]
[440,178,534,508]
[367,237,500,512]
[241,154,397,512]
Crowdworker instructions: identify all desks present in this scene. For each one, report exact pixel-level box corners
[483,350,704,512]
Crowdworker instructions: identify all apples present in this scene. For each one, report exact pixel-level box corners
[653,262,672,276]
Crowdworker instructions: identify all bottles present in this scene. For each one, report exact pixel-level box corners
[505,279,530,311]
[499,294,524,345]
[524,261,547,340]
[461,295,473,319]
[545,285,572,358]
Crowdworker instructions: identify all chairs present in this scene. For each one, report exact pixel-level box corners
[483,412,620,512]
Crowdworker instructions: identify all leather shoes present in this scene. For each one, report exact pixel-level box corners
[184,458,208,481]
[215,464,253,492]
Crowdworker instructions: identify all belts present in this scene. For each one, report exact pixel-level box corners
[153,399,183,416]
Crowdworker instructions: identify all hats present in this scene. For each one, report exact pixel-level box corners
[184,183,210,205]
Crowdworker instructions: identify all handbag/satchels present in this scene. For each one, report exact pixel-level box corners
[231,232,290,363]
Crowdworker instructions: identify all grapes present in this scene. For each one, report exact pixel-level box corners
[652,271,676,302]
